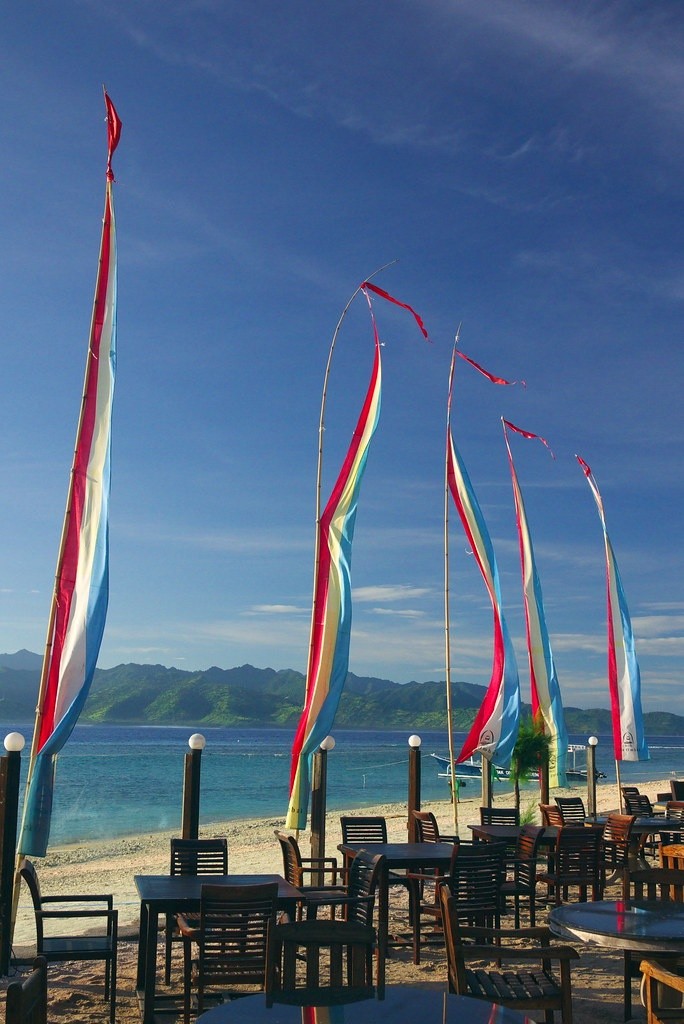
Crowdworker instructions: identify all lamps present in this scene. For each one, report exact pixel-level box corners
[408,735,422,750]
[4,732,25,757]
[588,736,598,747]
[320,736,336,750]
[189,733,205,754]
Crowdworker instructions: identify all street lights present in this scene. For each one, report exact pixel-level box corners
[407,735,427,844]
[310,735,336,887]
[586,736,599,818]
[182,733,208,877]
[0,731,26,981]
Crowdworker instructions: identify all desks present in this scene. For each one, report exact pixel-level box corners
[549,900,684,1024]
[585,816,682,884]
[337,843,485,944]
[134,874,305,1024]
[467,825,588,903]
[194,985,536,1024]
[653,800,684,811]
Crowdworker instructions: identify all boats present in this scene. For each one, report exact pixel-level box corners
[430,745,607,791]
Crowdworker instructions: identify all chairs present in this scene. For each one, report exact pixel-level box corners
[1,779,684,1024]
